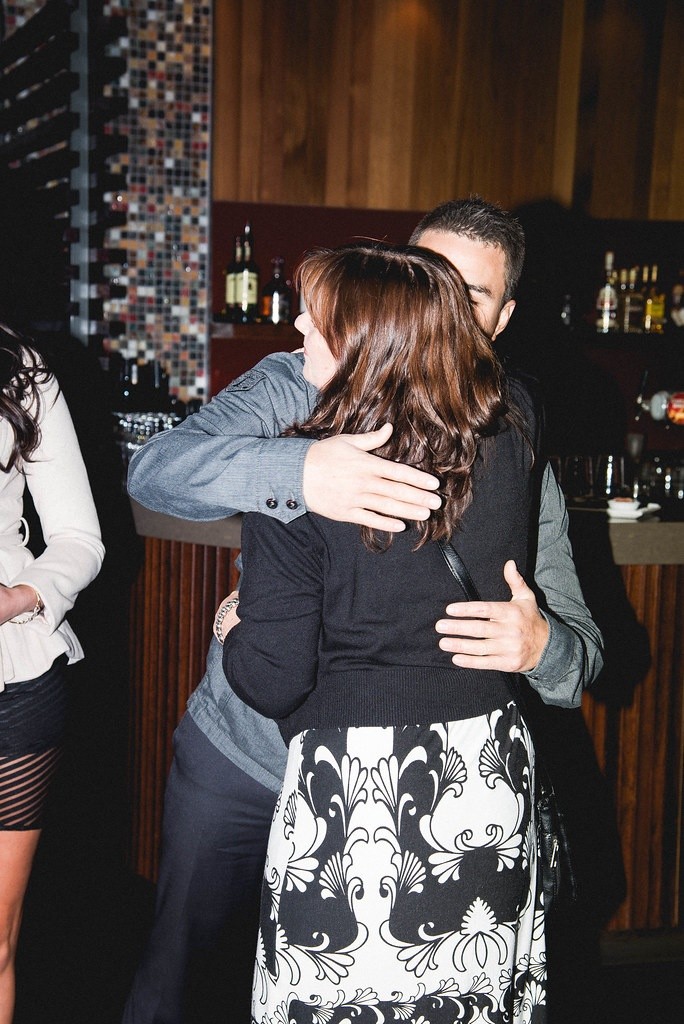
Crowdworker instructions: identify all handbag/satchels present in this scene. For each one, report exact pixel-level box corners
[528,703,629,963]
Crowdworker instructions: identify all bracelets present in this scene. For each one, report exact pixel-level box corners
[9,596,41,624]
[216,598,239,644]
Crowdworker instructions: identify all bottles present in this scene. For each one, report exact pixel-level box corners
[591,263,666,334]
[223,234,291,326]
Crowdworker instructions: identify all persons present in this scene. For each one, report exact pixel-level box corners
[123,195,605,1024]
[0,325,106,1024]
[215,237,548,1024]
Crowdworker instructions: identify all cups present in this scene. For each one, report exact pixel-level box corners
[545,451,684,502]
